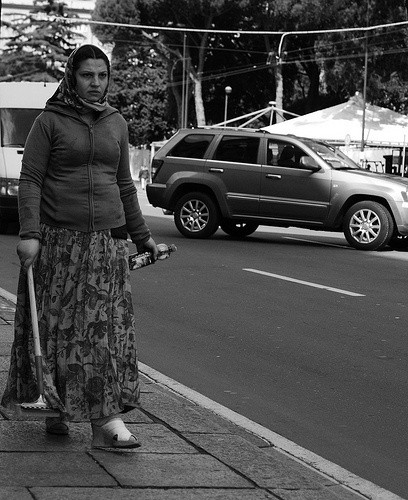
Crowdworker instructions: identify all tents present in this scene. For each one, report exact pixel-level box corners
[260,92,408,147]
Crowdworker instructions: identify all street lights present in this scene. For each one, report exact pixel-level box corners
[224,85,232,127]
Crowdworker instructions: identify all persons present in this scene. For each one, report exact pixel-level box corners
[365,163,372,171]
[139,164,149,191]
[0,45,161,450]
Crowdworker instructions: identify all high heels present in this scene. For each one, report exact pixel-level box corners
[92,418,141,448]
[45,410,69,435]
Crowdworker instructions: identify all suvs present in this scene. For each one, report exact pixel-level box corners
[145,125,408,251]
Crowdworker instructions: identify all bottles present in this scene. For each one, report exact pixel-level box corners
[128,243,177,270]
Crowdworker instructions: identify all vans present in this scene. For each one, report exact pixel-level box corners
[0,81,63,234]
